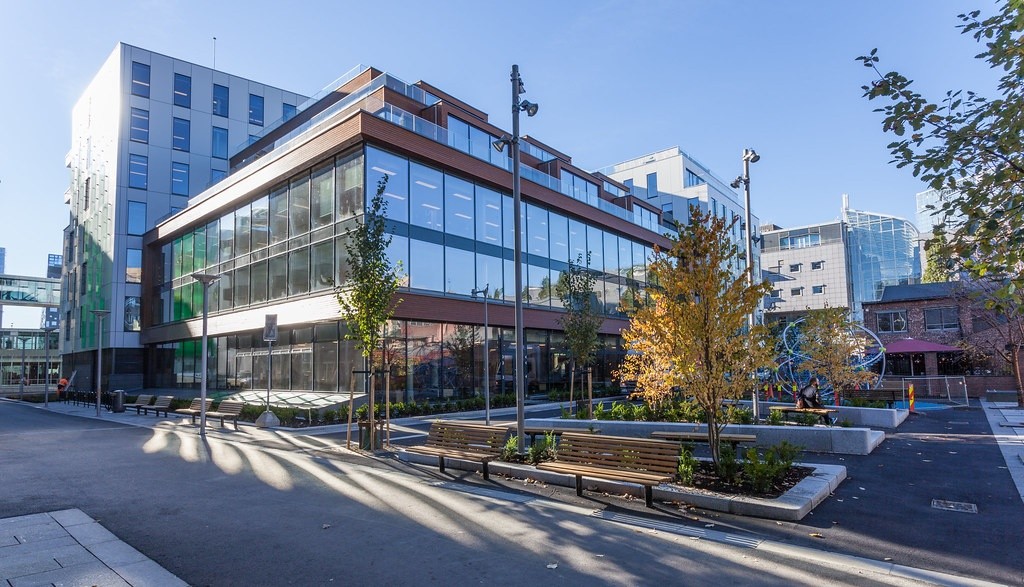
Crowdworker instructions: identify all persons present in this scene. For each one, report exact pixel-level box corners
[798,377,838,425]
[5,338,12,349]
[57,377,68,400]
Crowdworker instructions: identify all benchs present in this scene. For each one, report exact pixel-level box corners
[123,395,156,415]
[652,430,756,457]
[510,427,601,448]
[843,388,909,409]
[141,396,176,418]
[769,406,838,426]
[175,398,215,424]
[535,432,685,507]
[406,419,511,481]
[206,399,247,430]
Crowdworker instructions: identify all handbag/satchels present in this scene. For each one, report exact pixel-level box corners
[796,397,804,408]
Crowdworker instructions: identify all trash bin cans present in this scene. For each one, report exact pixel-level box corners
[112,389,126,413]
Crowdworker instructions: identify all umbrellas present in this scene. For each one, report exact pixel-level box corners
[856,336,962,376]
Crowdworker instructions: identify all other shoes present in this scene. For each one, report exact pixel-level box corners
[831,418,837,424]
